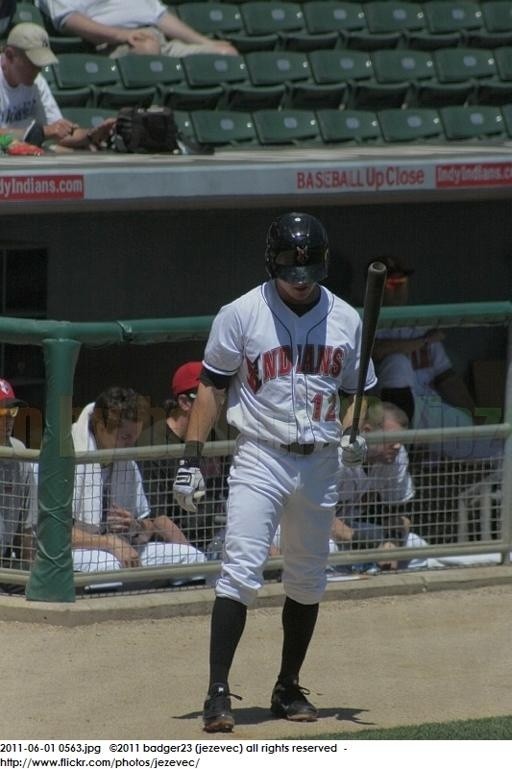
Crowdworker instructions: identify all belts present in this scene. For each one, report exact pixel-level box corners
[248,431,334,459]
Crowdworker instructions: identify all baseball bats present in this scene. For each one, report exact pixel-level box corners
[350,261,386,442]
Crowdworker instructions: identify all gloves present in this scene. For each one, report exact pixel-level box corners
[171,458,207,515]
[336,420,368,470]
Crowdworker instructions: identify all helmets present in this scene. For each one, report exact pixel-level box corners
[263,208,334,287]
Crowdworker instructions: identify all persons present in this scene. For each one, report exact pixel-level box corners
[0,379,38,594]
[133,361,230,591]
[36,0,238,55]
[32,388,208,594]
[0,22,116,155]
[271,405,444,573]
[262,251,479,460]
[173,213,379,731]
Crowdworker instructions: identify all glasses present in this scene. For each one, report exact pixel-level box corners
[0,405,20,418]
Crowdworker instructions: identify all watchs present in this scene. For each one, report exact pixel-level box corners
[178,458,199,468]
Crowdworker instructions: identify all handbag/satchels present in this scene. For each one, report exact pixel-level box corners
[108,98,217,156]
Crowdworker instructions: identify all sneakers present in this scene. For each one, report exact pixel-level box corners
[201,682,236,734]
[269,680,320,723]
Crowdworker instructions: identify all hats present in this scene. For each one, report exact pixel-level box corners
[169,359,205,399]
[0,377,27,412]
[367,253,413,283]
[5,21,59,68]
